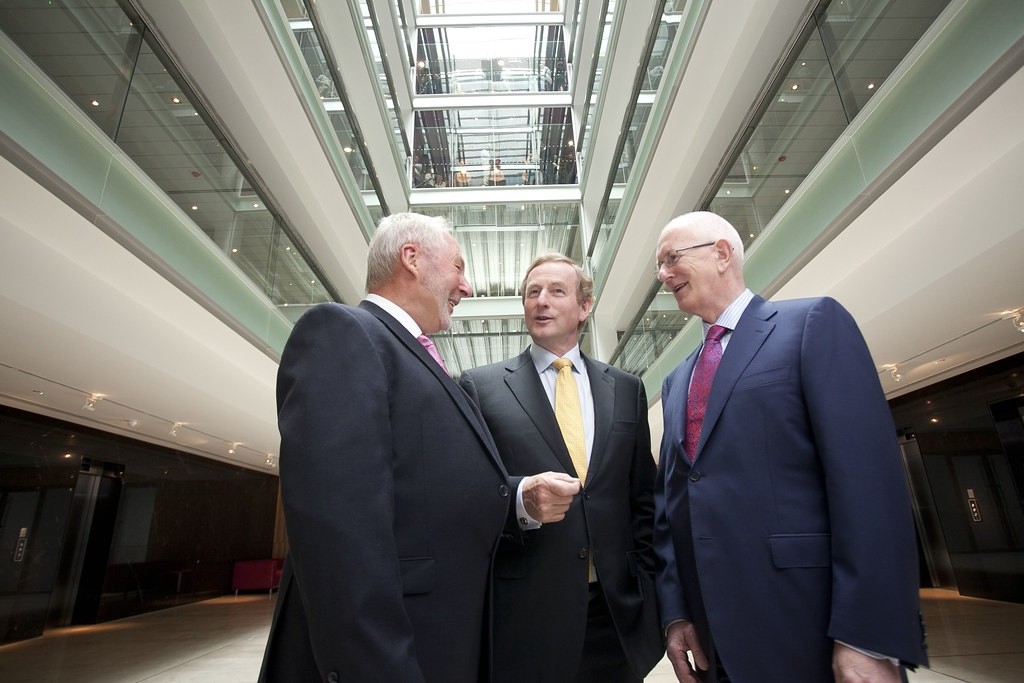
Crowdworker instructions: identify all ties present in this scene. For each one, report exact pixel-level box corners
[551,358,588,489]
[684,325,730,465]
[417,334,451,381]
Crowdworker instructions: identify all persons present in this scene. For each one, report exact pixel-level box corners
[566,152,577,183]
[539,61,567,91]
[648,58,665,87]
[461,252,665,683]
[413,154,448,188]
[656,212,930,683]
[258,214,510,683]
[317,69,333,98]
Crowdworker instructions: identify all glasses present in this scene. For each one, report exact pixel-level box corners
[657,242,734,269]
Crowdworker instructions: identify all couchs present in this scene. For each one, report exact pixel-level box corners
[232,558,284,594]
